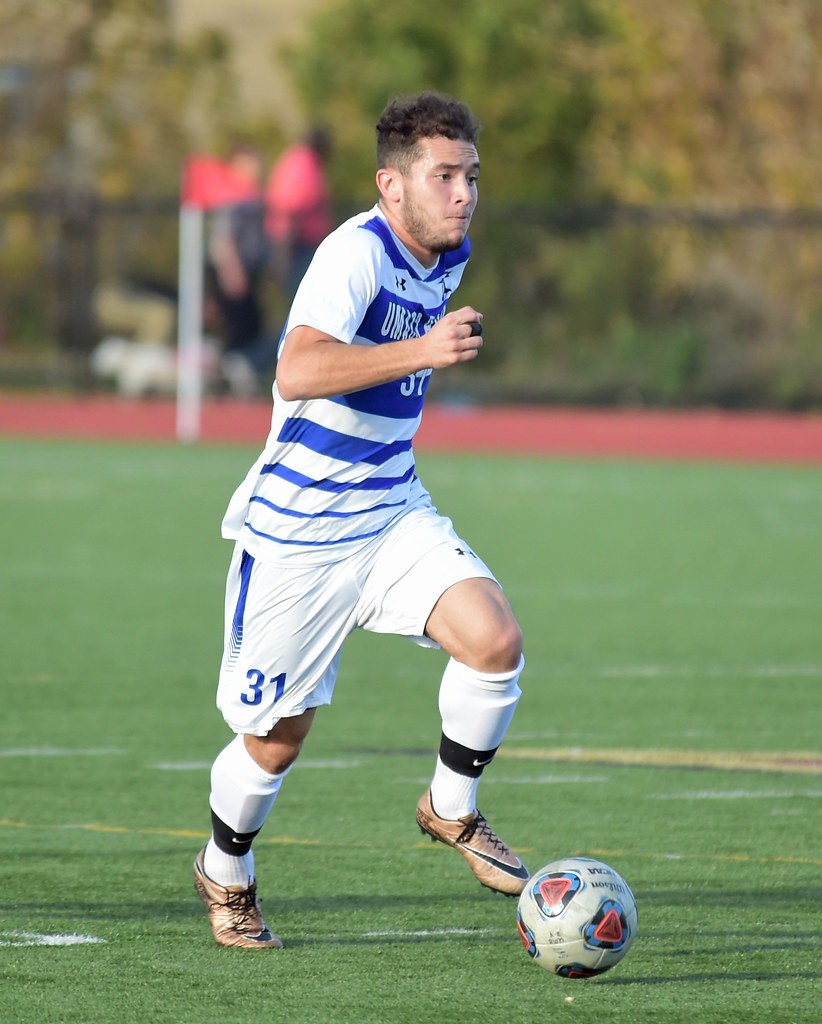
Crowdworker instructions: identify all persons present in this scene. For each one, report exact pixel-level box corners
[194,95,533,954]
[206,117,333,396]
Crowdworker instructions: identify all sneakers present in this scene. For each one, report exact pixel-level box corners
[192,844,283,949]
[416,785,531,897]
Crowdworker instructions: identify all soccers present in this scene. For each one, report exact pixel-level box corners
[513,856,640,978]
